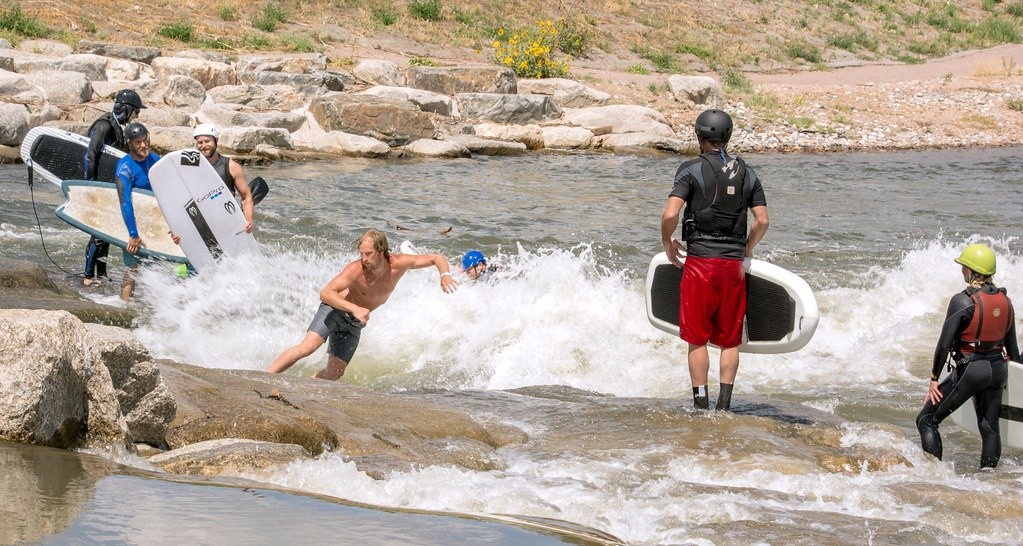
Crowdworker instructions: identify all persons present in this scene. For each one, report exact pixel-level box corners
[462,251,498,286]
[915,244,1020,469]
[115,122,160,301]
[167,123,254,280]
[82,88,148,287]
[662,109,769,412]
[266,228,459,381]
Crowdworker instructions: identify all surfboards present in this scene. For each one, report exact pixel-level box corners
[942,356,1023,452]
[55,181,188,264]
[644,251,820,353]
[146,147,258,277]
[19,124,127,186]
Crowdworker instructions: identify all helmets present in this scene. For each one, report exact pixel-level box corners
[114,89,148,110]
[953,243,997,275]
[123,122,149,144]
[694,110,733,142]
[462,250,484,273]
[193,123,219,141]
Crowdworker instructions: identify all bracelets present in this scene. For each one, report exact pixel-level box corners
[440,272,450,278]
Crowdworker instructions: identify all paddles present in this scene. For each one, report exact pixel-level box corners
[241,176,269,216]
